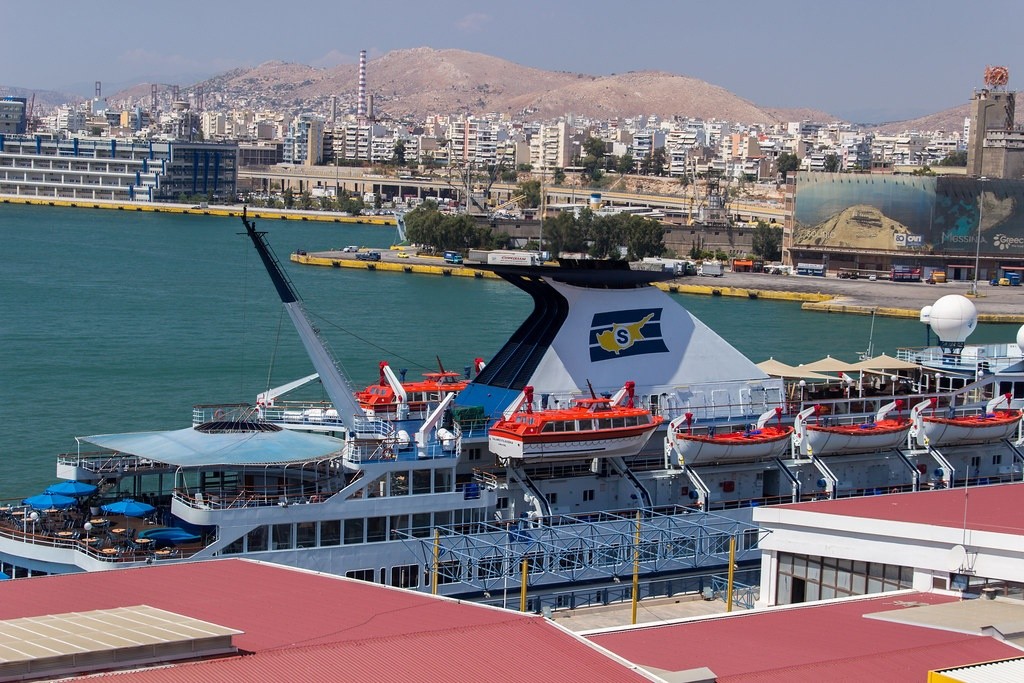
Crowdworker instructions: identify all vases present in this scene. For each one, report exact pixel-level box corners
[90,506,100,516]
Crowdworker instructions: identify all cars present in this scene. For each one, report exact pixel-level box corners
[990,279,999,286]
[398,251,409,258]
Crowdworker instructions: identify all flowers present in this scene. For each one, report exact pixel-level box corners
[90,496,101,507]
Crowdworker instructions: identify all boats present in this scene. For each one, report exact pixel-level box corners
[673,425,795,464]
[917,409,1022,446]
[354,357,487,423]
[800,418,914,455]
[489,397,666,465]
[0,209,1024,614]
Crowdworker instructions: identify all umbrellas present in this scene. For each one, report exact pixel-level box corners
[100,498,155,538]
[21,492,78,509]
[46,480,97,496]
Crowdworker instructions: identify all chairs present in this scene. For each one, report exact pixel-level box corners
[0,504,179,563]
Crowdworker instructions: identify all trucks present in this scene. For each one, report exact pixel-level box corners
[643,254,697,278]
[697,261,724,277]
[343,245,359,252]
[444,251,463,264]
[998,272,1020,286]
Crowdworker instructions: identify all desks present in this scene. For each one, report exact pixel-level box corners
[81,538,97,547]
[103,549,116,561]
[89,519,107,534]
[0,508,9,518]
[21,518,32,532]
[112,529,126,540]
[11,511,25,525]
[135,539,151,551]
[154,550,171,558]
[43,510,59,516]
[53,531,74,539]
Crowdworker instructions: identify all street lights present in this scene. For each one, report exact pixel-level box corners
[974,177,990,294]
